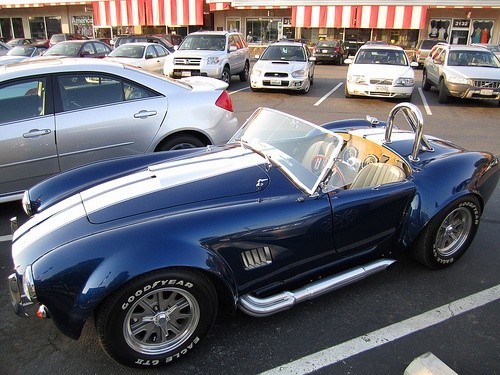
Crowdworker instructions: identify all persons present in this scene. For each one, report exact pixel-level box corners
[438,26,446,39]
[480,28,489,43]
[247,31,257,43]
[432,27,437,35]
[473,27,482,43]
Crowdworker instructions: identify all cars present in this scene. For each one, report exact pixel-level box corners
[0,33,183,99]
[470,43,500,61]
[250,41,316,95]
[312,38,349,66]
[360,40,389,56]
[1,57,241,203]
[343,44,419,102]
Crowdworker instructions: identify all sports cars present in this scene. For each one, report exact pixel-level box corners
[7,101,500,370]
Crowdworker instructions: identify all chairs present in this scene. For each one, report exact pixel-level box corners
[349,163,406,189]
[302,141,339,183]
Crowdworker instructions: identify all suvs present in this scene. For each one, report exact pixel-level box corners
[162,28,251,90]
[422,42,500,105]
[414,38,449,69]
